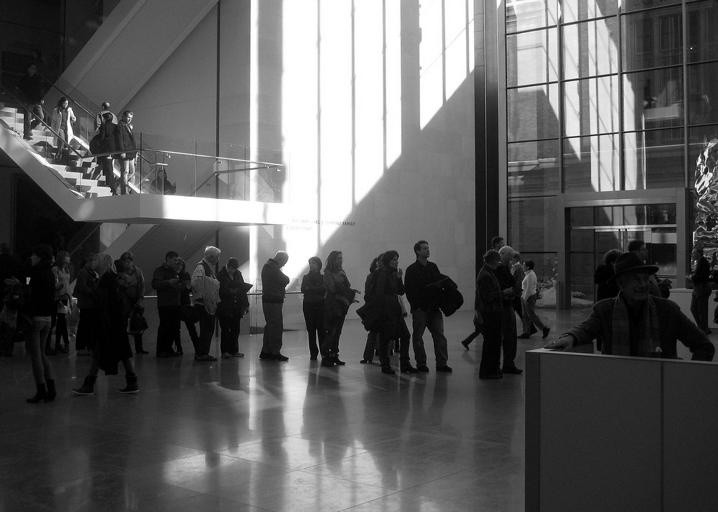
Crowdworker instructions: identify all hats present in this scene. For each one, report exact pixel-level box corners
[498,244,520,261]
[613,251,658,275]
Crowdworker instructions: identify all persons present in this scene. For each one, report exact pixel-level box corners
[259,253,289,361]
[92,112,119,195]
[114,110,139,194]
[23,63,44,140]
[301,251,355,366]
[1,241,148,402]
[360,240,452,374]
[544,239,716,362]
[461,238,550,379]
[49,96,77,159]
[151,246,248,362]
[94,101,118,132]
[151,167,177,194]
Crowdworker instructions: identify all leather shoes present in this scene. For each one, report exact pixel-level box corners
[136,350,150,354]
[517,326,550,339]
[479,367,523,379]
[460,341,469,350]
[379,361,452,374]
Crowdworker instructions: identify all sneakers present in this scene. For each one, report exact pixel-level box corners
[118,386,139,394]
[157,350,184,358]
[310,349,319,360]
[194,353,217,362]
[72,387,96,396]
[221,352,244,357]
[259,353,288,361]
[45,344,70,354]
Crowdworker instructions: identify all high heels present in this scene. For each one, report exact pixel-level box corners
[360,357,372,364]
[322,357,345,367]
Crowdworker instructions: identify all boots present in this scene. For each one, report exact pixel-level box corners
[26,379,57,405]
